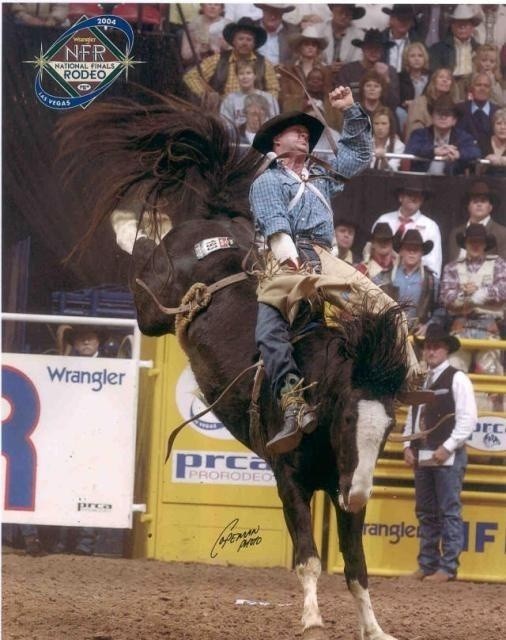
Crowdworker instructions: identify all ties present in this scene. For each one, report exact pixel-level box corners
[394,217,414,237]
[424,371,436,394]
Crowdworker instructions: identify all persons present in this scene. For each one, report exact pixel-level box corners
[336,216,369,264]
[400,43,433,103]
[471,44,502,79]
[481,104,505,170]
[439,223,505,376]
[392,230,437,328]
[369,110,407,173]
[406,69,455,136]
[334,28,403,106]
[353,71,390,109]
[380,5,423,69]
[9,3,73,30]
[457,75,501,138]
[286,68,337,127]
[405,96,481,175]
[185,2,231,57]
[248,4,302,65]
[216,60,277,146]
[229,96,272,141]
[292,99,342,166]
[429,4,483,76]
[279,26,332,118]
[438,180,506,272]
[368,174,442,307]
[403,323,478,582]
[184,18,278,109]
[314,3,368,62]
[249,86,377,453]
[364,224,396,294]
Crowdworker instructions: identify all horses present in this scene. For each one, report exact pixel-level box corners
[53,81,436,634]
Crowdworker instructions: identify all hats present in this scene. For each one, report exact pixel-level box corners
[448,5,483,27]
[455,224,497,252]
[397,229,438,260]
[366,223,400,243]
[67,323,111,343]
[286,26,329,50]
[385,176,435,200]
[221,17,267,49]
[325,4,365,20]
[251,109,325,160]
[382,4,424,20]
[462,181,499,211]
[418,323,460,355]
[351,28,396,49]
[253,2,295,12]
[424,93,465,116]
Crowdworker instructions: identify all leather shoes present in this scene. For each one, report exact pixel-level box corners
[262,398,317,463]
[407,564,455,586]
[392,379,432,414]
